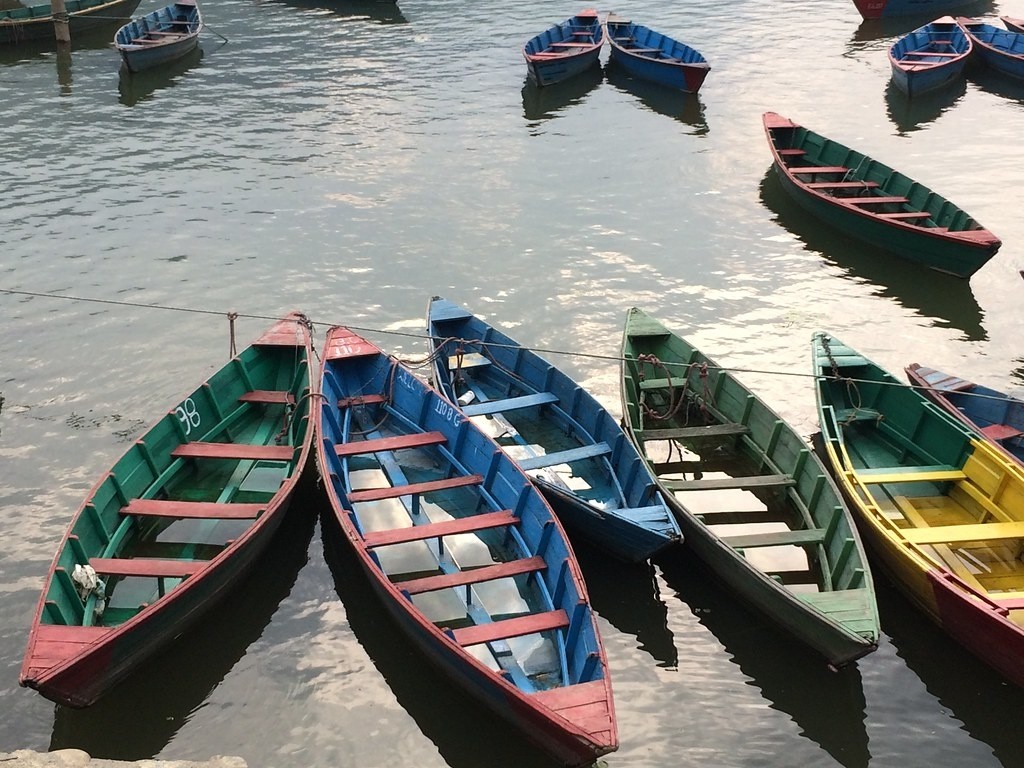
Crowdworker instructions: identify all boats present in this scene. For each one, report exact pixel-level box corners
[0,0,142,43]
[427,294,687,578]
[606,10,711,94]
[812,331,1024,678]
[955,15,1024,82]
[619,305,882,674]
[903,362,1024,479]
[762,110,1003,279]
[887,15,973,98]
[521,6,605,89]
[314,324,619,768]
[19,309,316,711]
[113,0,203,72]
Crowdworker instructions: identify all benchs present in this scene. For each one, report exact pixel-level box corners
[835,407,880,422]
[898,521,1024,544]
[158,21,194,24]
[451,609,570,646]
[642,424,752,441]
[838,196,909,204]
[571,32,594,35]
[928,40,952,44]
[363,509,521,548]
[805,182,880,188]
[144,31,189,36]
[613,37,636,40]
[780,149,807,156]
[638,377,690,389]
[516,441,613,471]
[461,391,560,417]
[853,466,968,485]
[788,166,849,173]
[659,473,797,491]
[334,430,448,456]
[171,441,294,460]
[448,352,492,370]
[904,52,961,57]
[995,597,1024,610]
[129,39,164,43]
[898,60,937,65]
[338,394,384,407]
[536,53,570,55]
[548,42,594,47]
[392,554,547,594]
[876,212,931,219]
[627,49,665,52]
[720,529,828,548]
[663,58,681,62]
[89,556,209,599]
[239,389,296,403]
[345,474,485,503]
[929,227,949,233]
[980,422,1024,441]
[119,498,269,518]
